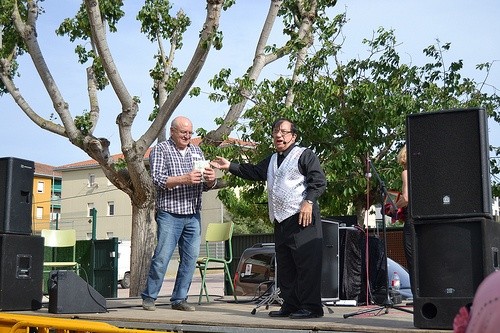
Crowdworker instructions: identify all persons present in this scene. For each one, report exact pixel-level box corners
[389,144,414,305]
[209,118,327,318]
[141,116,217,312]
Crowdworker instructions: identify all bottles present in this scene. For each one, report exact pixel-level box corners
[391,272,400,291]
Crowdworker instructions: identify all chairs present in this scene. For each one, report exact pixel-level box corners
[177,221,239,306]
[40,229,80,308]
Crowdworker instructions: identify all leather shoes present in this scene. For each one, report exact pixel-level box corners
[269,308,291,317]
[289,309,322,318]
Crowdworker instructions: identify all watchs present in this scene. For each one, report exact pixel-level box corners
[307,200,313,204]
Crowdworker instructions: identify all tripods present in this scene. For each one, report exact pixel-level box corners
[342,168,413,320]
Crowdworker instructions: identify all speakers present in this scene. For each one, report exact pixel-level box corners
[48,269,106,314]
[0,234,45,312]
[319,221,339,301]
[406,107,493,220]
[408,220,500,329]
[0,156,35,234]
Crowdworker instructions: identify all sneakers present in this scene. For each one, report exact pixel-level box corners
[172,300,196,311]
[142,297,156,311]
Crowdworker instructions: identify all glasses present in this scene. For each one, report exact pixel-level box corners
[173,127,194,137]
[273,129,294,137]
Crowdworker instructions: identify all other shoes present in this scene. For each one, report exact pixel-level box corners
[406,302,413,306]
